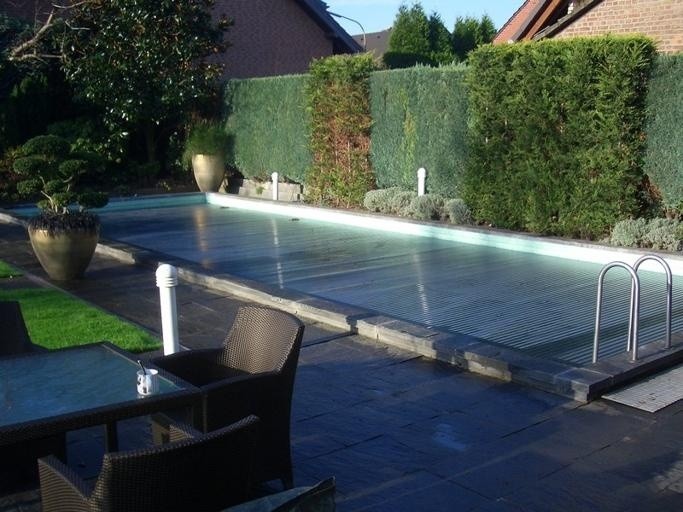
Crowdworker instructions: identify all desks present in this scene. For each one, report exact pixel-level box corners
[0,341,205,484]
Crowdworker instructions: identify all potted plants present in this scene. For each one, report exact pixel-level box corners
[188,119,234,194]
[12,135,109,288]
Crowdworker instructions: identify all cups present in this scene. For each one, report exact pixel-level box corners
[135,368,159,398]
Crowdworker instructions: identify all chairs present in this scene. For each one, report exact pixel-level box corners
[149,305,308,491]
[36,409,270,512]
[0,299,52,355]
